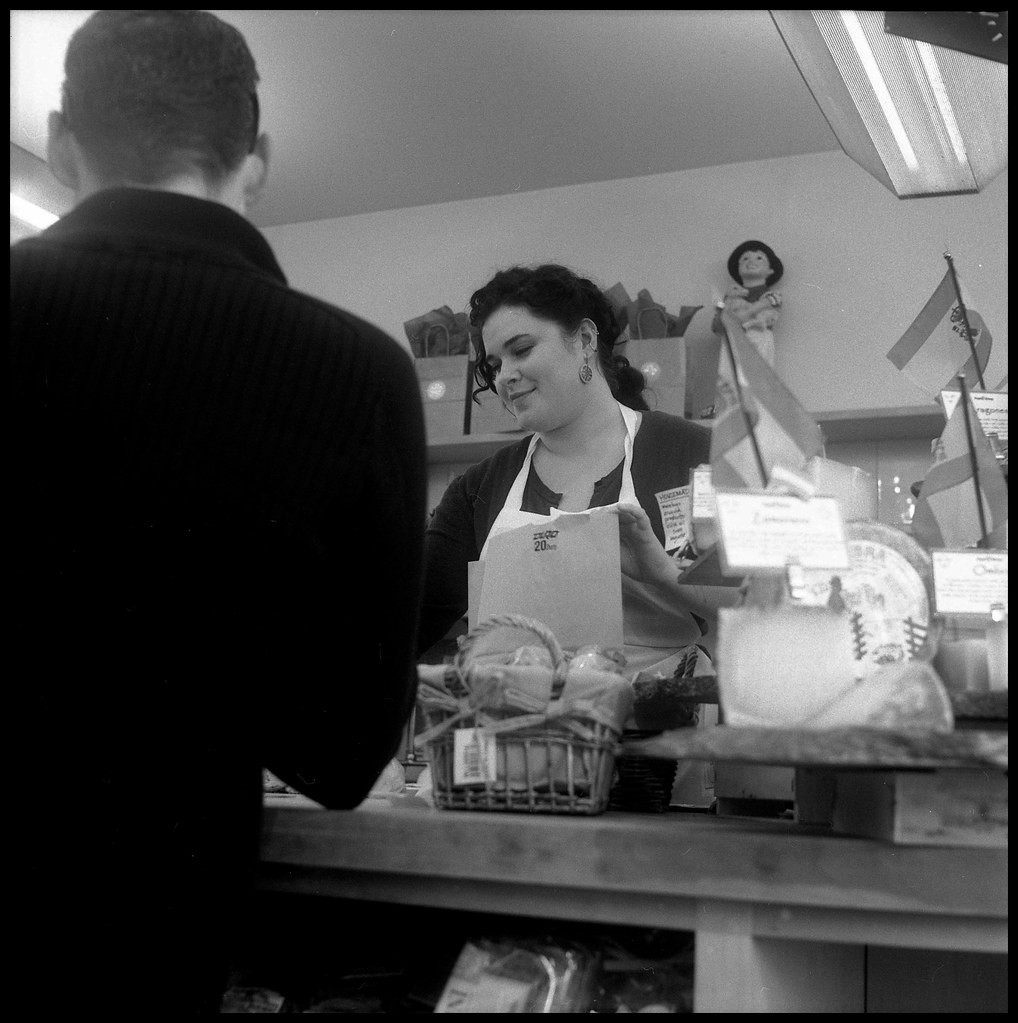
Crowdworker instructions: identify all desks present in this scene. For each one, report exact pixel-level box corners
[264,792,1008,1011]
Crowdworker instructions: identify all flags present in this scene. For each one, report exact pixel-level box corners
[887,263,993,406]
[709,305,828,493]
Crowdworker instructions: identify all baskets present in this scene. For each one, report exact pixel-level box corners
[428,611,628,814]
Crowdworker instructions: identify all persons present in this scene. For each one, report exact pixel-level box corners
[7,7,427,1022]
[426,261,748,768]
[722,240,783,373]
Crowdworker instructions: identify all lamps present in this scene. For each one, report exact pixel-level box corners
[771,9,1007,198]
